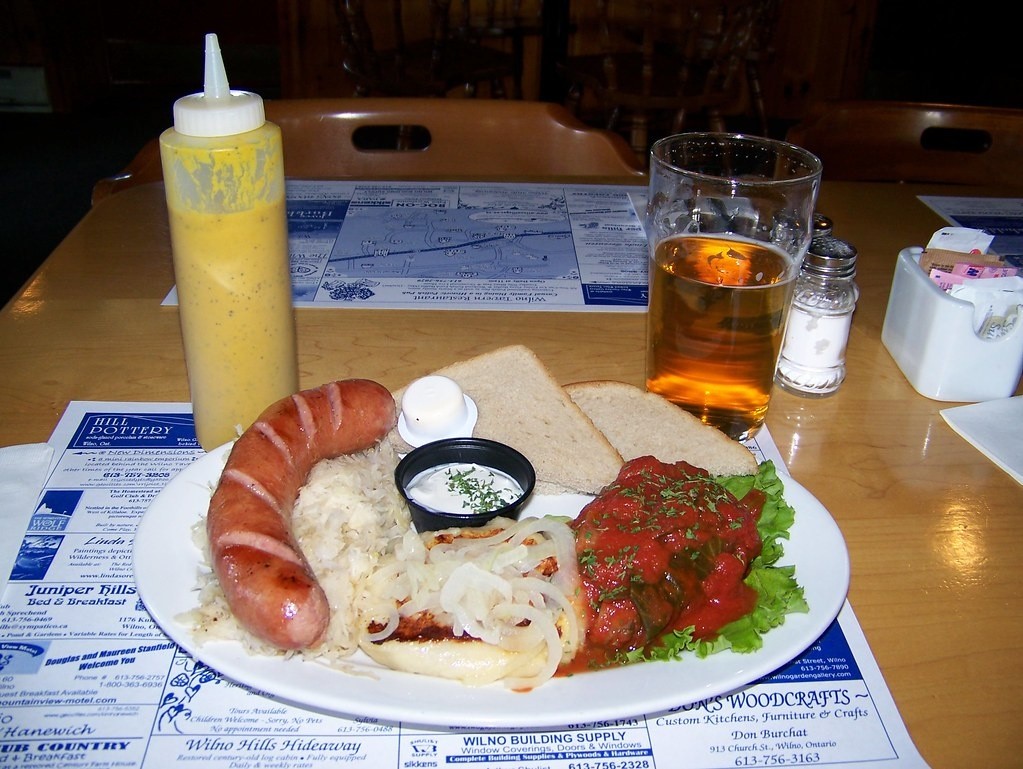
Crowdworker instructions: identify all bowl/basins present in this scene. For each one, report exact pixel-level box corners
[395,437,536,533]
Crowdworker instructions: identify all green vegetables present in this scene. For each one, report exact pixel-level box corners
[443,467,525,515]
[568,465,746,618]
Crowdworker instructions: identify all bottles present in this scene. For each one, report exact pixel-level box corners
[813,212,832,242]
[774,236,856,397]
[159,33,297,453]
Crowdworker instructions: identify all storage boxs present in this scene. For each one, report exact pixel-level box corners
[880,244,1023,405]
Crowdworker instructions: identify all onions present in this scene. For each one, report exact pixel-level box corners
[359,514,583,691]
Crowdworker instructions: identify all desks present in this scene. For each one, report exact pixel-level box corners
[2,179,1022,769]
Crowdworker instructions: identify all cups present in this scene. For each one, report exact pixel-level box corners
[646,132,823,440]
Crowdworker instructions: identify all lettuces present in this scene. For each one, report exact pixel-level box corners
[619,457,810,667]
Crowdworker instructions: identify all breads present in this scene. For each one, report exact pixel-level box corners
[399,347,760,493]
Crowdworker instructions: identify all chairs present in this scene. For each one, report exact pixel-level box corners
[262,94,643,180]
[776,103,1022,190]
[330,0,771,167]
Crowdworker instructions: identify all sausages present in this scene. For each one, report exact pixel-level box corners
[207,378,396,652]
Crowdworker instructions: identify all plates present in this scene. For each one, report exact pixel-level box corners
[132,441,850,728]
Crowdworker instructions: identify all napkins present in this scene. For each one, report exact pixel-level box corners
[939,394,1023,485]
